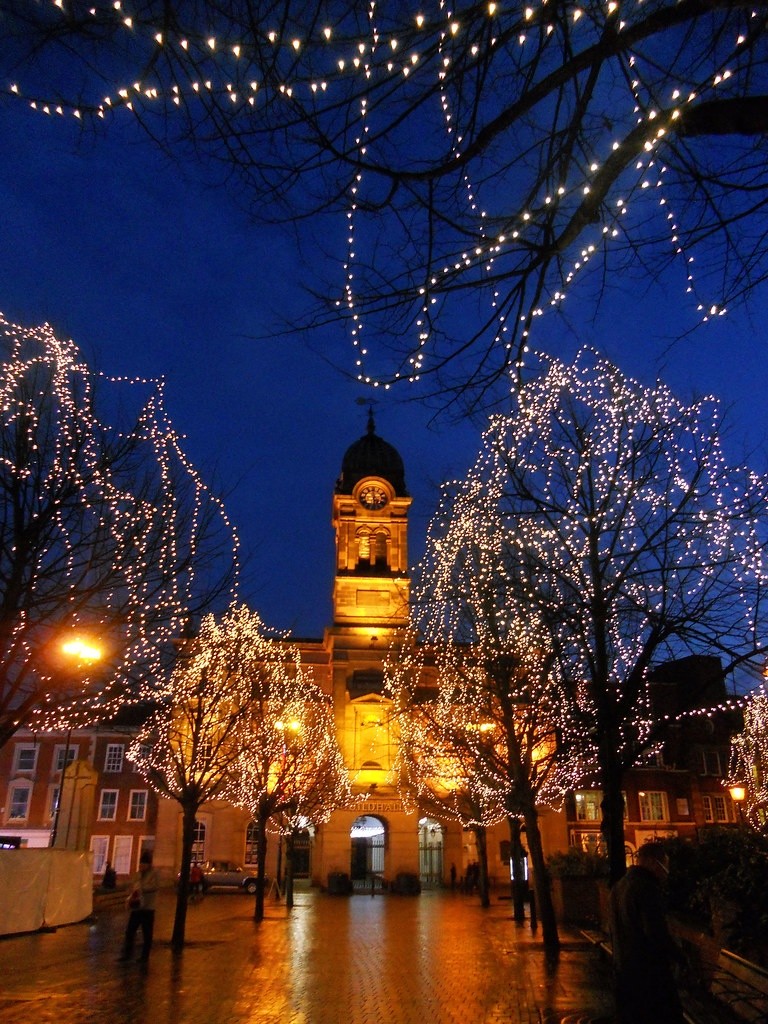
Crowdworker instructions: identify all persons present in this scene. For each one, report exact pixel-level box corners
[188,860,202,895]
[471,858,481,890]
[463,858,473,893]
[450,862,457,890]
[113,851,158,976]
[102,861,116,889]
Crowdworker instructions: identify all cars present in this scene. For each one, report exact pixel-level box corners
[198,860,267,894]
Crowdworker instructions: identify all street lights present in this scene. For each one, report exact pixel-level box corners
[275,720,300,898]
[729,786,745,824]
[51,640,100,848]
